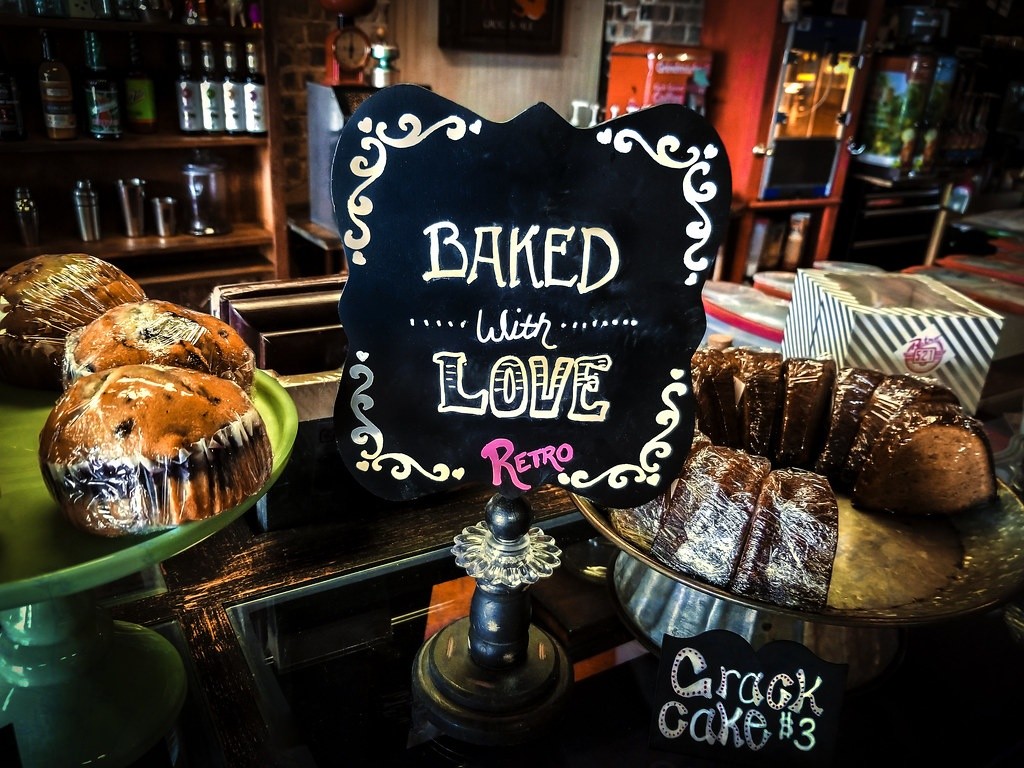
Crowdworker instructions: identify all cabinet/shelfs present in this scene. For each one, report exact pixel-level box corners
[0,0,289,291]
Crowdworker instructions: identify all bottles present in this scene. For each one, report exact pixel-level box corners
[72,179,101,243]
[180,150,234,237]
[0,0,272,138]
[16,187,43,248]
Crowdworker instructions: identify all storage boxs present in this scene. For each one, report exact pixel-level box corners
[781,269,1005,417]
[522,565,639,665]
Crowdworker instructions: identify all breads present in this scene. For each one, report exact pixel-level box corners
[609,346,997,611]
[0,252,275,537]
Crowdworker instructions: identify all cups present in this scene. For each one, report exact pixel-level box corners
[153,197,179,237]
[118,177,146,237]
